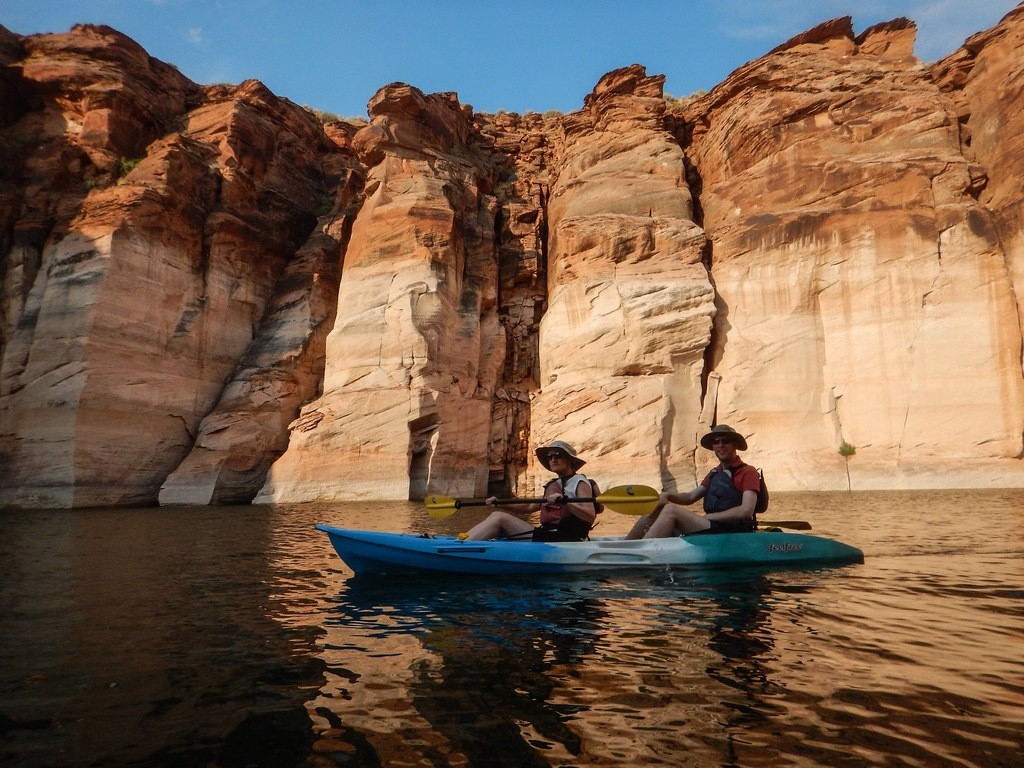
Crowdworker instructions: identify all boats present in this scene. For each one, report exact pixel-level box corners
[313,522,864,582]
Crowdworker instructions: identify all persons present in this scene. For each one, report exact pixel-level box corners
[456,441,604,542]
[623,424,769,540]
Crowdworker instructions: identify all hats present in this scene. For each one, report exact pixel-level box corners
[700,425,748,451]
[536,440,587,473]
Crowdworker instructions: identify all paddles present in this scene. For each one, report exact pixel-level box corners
[424,484,661,520]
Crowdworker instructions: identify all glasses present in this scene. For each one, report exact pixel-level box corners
[545,452,564,461]
[711,438,732,445]
